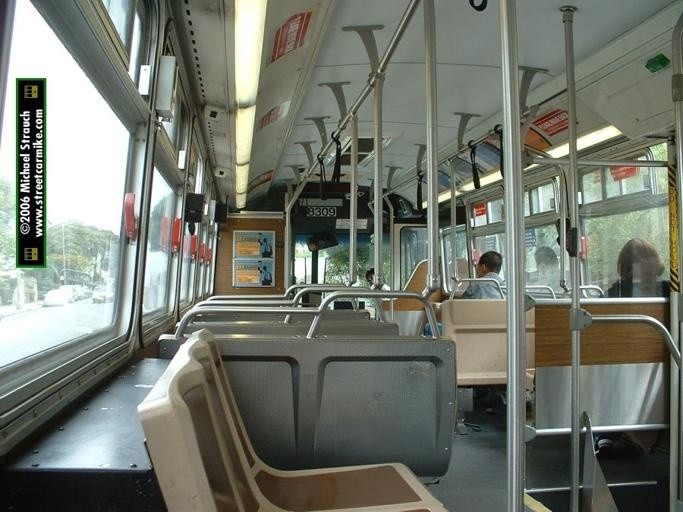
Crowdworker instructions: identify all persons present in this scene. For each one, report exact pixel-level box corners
[366,266,391,299]
[257,237,272,257]
[424,251,505,338]
[590,239,671,460]
[257,265,271,285]
[524,247,564,293]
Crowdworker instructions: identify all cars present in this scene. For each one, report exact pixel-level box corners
[40,284,113,309]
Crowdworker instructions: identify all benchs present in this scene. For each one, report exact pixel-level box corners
[136,284,683,512]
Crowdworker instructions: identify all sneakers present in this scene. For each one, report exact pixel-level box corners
[484,403,493,412]
[595,436,612,454]
